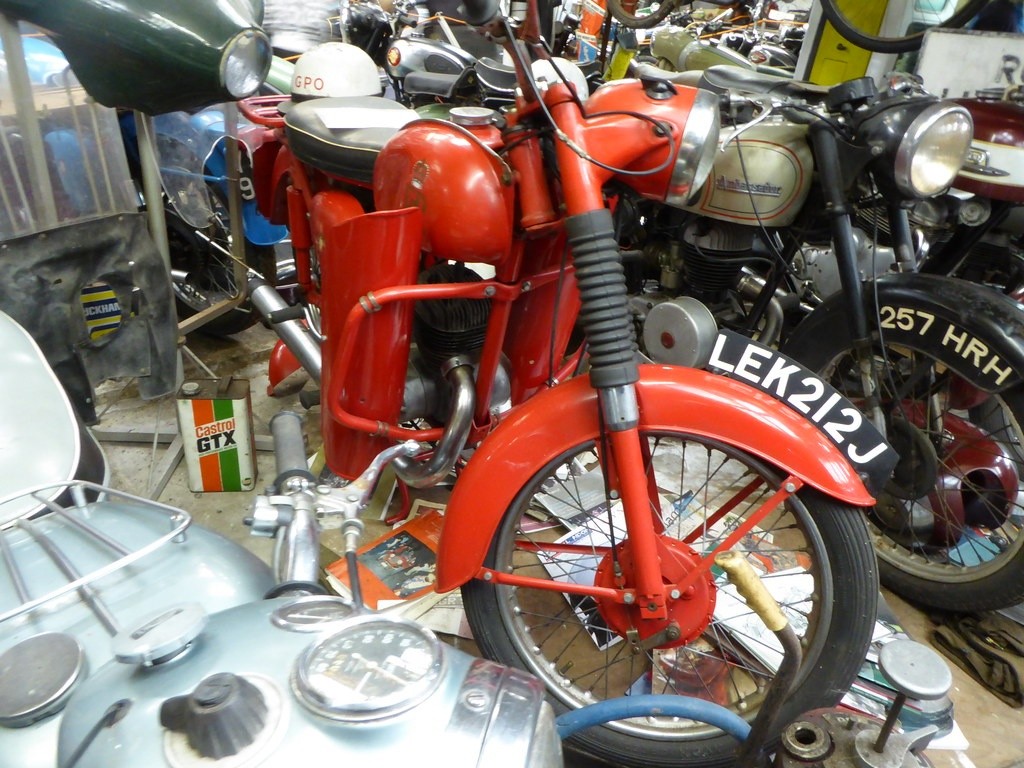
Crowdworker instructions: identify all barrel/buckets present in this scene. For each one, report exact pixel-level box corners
[176,376,257,492]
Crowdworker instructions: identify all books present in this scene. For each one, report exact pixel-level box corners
[322,507,463,622]
[532,449,977,768]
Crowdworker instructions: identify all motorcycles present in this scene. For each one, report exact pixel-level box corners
[1,0,1023,768]
